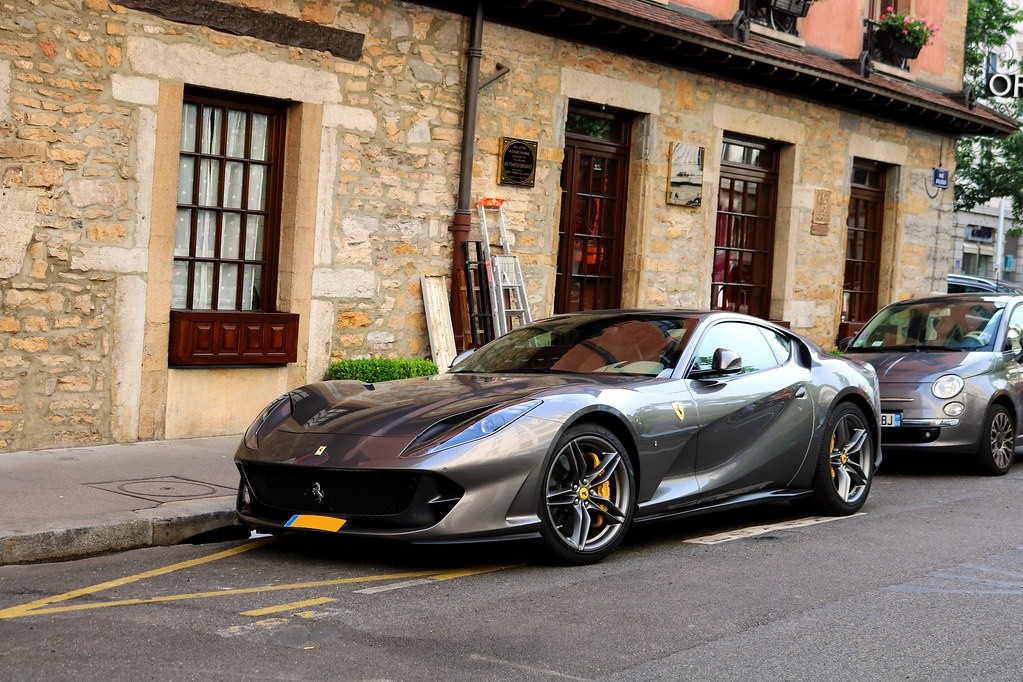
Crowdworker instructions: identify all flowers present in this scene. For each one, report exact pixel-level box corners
[879,6,943,48]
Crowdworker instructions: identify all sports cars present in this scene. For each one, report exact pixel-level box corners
[233,307,883,566]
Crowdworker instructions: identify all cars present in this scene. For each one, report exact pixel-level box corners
[838,291,1023,477]
[946,273,1023,295]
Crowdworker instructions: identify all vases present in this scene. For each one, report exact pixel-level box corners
[756,0,813,18]
[874,30,923,60]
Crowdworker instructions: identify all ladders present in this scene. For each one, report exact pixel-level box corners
[476,197,538,349]
[462,239,492,348]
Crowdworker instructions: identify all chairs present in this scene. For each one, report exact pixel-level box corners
[928,316,955,345]
